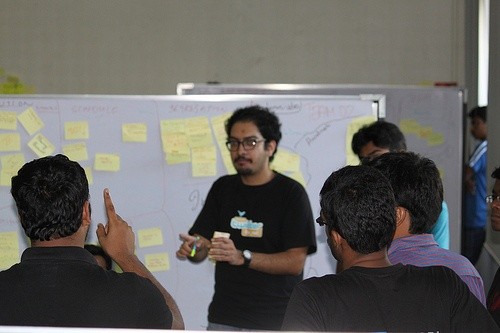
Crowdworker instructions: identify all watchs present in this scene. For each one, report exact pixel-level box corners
[239,249,252,269]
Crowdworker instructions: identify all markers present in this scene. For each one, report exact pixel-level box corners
[191,236,200,257]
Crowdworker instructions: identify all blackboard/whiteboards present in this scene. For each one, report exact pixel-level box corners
[176,81,463,255]
[0,93,388,330]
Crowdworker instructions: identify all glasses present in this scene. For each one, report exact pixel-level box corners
[226,137,269,152]
[485,195,500,203]
[316,216,334,236]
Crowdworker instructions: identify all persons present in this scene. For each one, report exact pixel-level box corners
[0,154,186,330]
[351,121,450,250]
[281,166,500,333]
[362,151,486,309]
[462,105,488,267]
[486,168,500,327]
[175,104,317,332]
